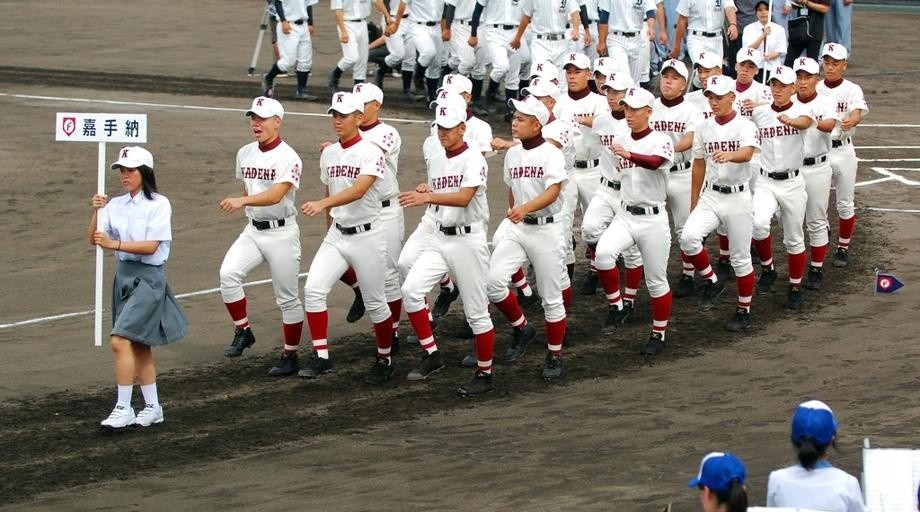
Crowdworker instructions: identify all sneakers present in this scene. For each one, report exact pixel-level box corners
[673,244,849,333]
[297,350,334,378]
[224,327,256,357]
[100,403,135,428]
[515,244,666,356]
[503,323,536,362]
[541,351,563,381]
[456,369,494,397]
[268,353,299,378]
[346,281,459,384]
[133,404,164,427]
[265,60,428,110]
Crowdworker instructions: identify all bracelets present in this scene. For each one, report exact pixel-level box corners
[115,240,122,250]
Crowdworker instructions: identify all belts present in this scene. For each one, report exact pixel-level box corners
[665,137,850,195]
[574,159,659,216]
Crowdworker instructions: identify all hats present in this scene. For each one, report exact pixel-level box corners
[352,82,384,104]
[686,451,747,489]
[754,0,773,9]
[110,146,154,170]
[327,92,365,115]
[245,96,283,122]
[791,400,836,445]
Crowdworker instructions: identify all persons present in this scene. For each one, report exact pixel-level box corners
[300,42,875,398]
[215,97,304,374]
[84,145,181,431]
[763,395,866,511]
[688,444,753,512]
[262,0,852,124]
